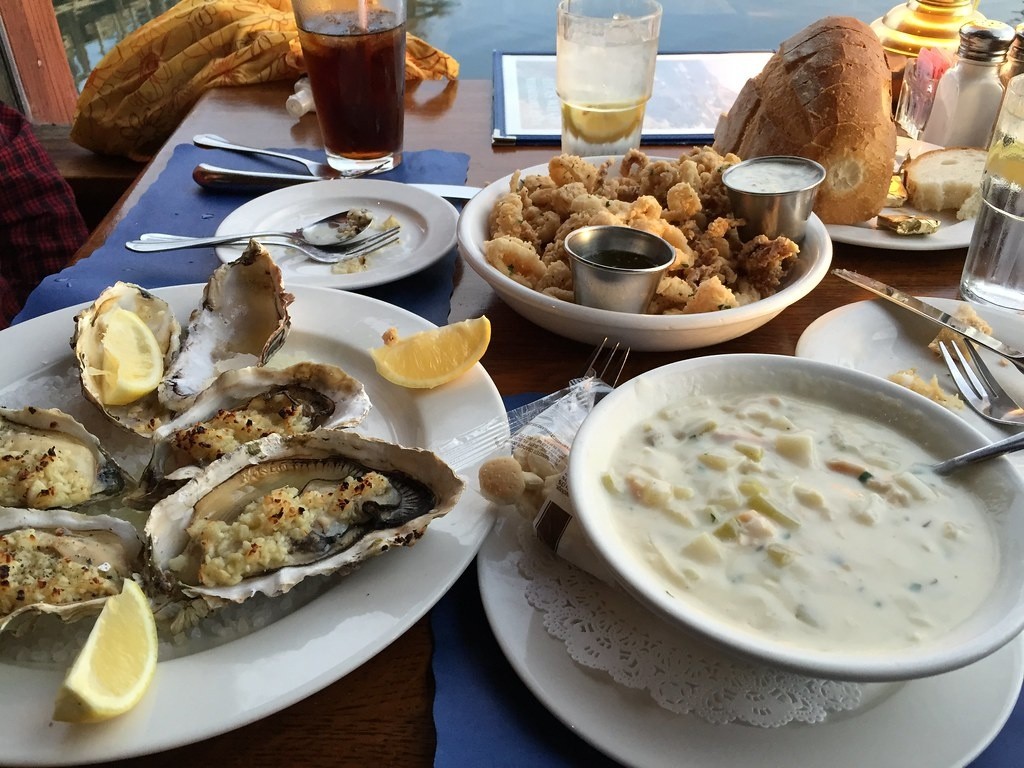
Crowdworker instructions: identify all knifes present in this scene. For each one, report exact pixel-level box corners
[192,163,483,199]
[832,268,1024,374]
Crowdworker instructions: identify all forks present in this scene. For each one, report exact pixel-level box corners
[582,337,630,389]
[140,226,400,263]
[193,134,390,178]
[938,338,1024,426]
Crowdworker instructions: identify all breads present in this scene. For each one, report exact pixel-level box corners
[927,302,992,364]
[712,16,989,235]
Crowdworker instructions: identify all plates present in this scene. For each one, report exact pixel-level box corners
[795,296,1024,476]
[477,506,1024,768]
[0,283,510,768]
[822,136,977,251]
[214,179,461,288]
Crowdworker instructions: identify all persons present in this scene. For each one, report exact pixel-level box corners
[0,97,90,332]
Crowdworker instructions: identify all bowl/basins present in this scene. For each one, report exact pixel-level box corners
[564,225,675,313]
[722,155,826,244]
[457,155,834,351]
[568,353,1024,679]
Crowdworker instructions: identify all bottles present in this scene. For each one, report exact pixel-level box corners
[998,33,1024,88]
[919,19,1016,151]
[286,76,317,117]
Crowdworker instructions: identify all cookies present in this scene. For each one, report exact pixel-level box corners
[479,435,570,521]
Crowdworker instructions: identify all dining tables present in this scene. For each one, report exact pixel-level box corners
[0,0,1024,768]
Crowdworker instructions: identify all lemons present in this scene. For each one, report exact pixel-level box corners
[52,578,158,724]
[366,315,490,387]
[99,309,163,405]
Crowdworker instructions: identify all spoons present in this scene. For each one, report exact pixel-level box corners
[125,209,374,252]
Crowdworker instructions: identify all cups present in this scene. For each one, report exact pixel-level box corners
[292,0,407,174]
[557,0,662,157]
[959,73,1024,315]
